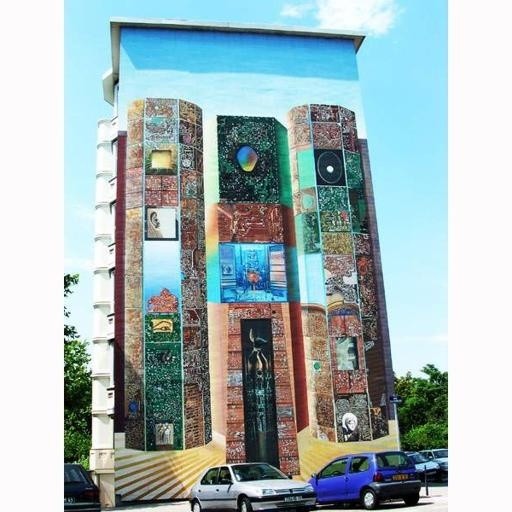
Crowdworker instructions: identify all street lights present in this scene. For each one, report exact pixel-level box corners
[389,393,404,468]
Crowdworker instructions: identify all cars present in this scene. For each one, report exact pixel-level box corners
[303,449,420,509]
[401,451,442,483]
[64,462,102,512]
[420,448,448,480]
[187,460,318,512]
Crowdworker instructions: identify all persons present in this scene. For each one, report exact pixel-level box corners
[341,412,360,442]
